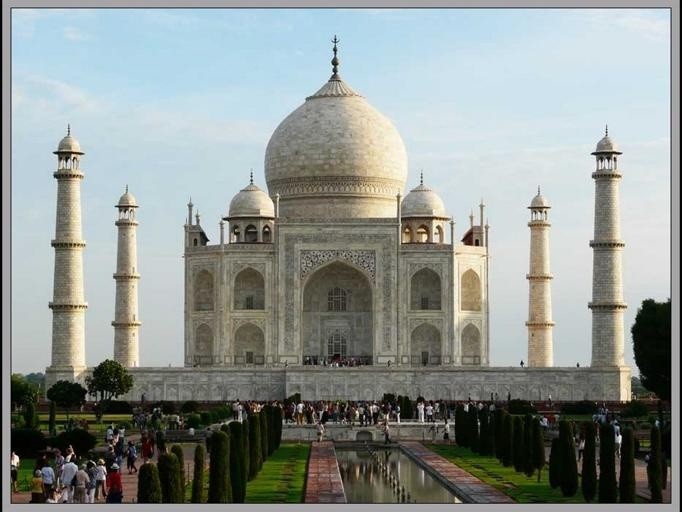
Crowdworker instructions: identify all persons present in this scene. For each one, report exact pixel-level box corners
[441,420,449,439]
[319,358,348,367]
[232,398,496,425]
[428,420,437,440]
[539,398,623,462]
[317,422,324,443]
[11,406,228,504]
[383,422,393,444]
